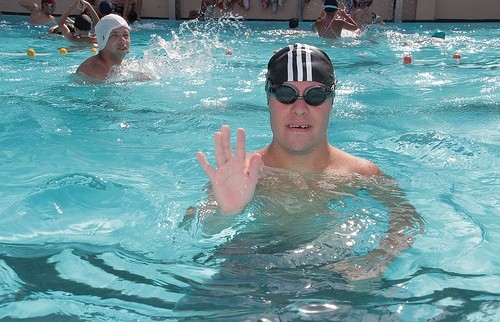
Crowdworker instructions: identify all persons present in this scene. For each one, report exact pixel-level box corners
[29,0,150,81]
[197,43,424,281]
[289,0,384,39]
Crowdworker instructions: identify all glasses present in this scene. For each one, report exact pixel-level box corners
[42,3,57,9]
[265,78,336,106]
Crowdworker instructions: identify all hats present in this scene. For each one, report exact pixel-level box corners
[99,1,113,15]
[288,17,299,27]
[95,14,131,52]
[74,14,92,31]
[323,0,338,10]
[42,0,56,4]
[266,43,336,88]
